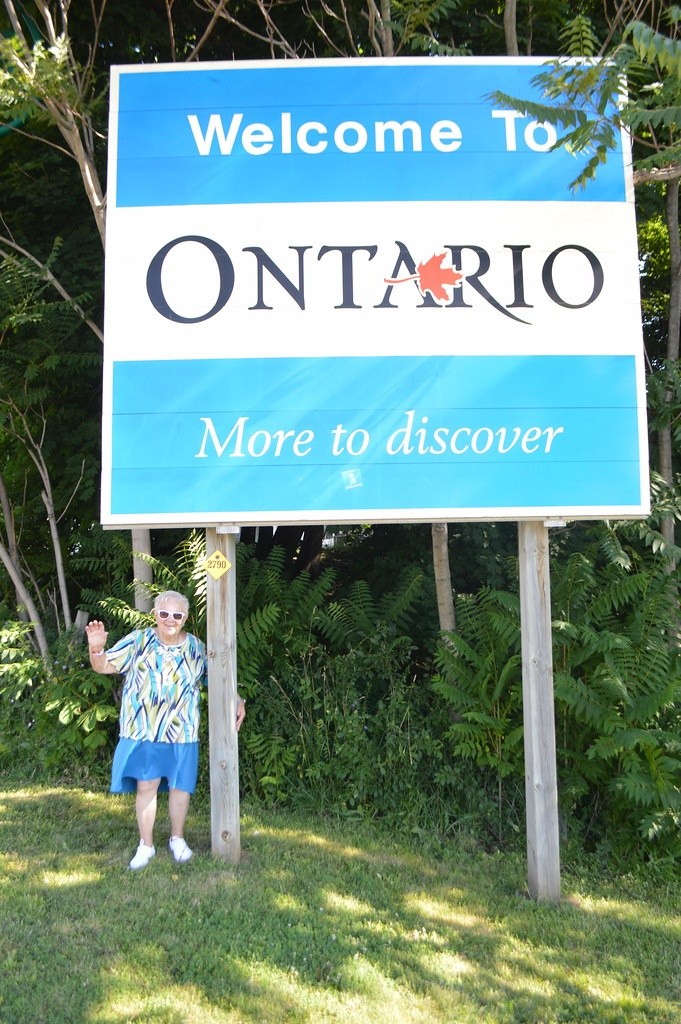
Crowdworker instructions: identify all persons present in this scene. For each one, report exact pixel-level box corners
[85,591,246,868]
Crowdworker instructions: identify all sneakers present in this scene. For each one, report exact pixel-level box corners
[130,838,155,869]
[169,835,193,861]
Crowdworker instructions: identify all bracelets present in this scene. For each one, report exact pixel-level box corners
[237,698,246,703]
[92,649,104,656]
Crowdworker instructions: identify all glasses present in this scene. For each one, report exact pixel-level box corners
[154,610,187,621]
